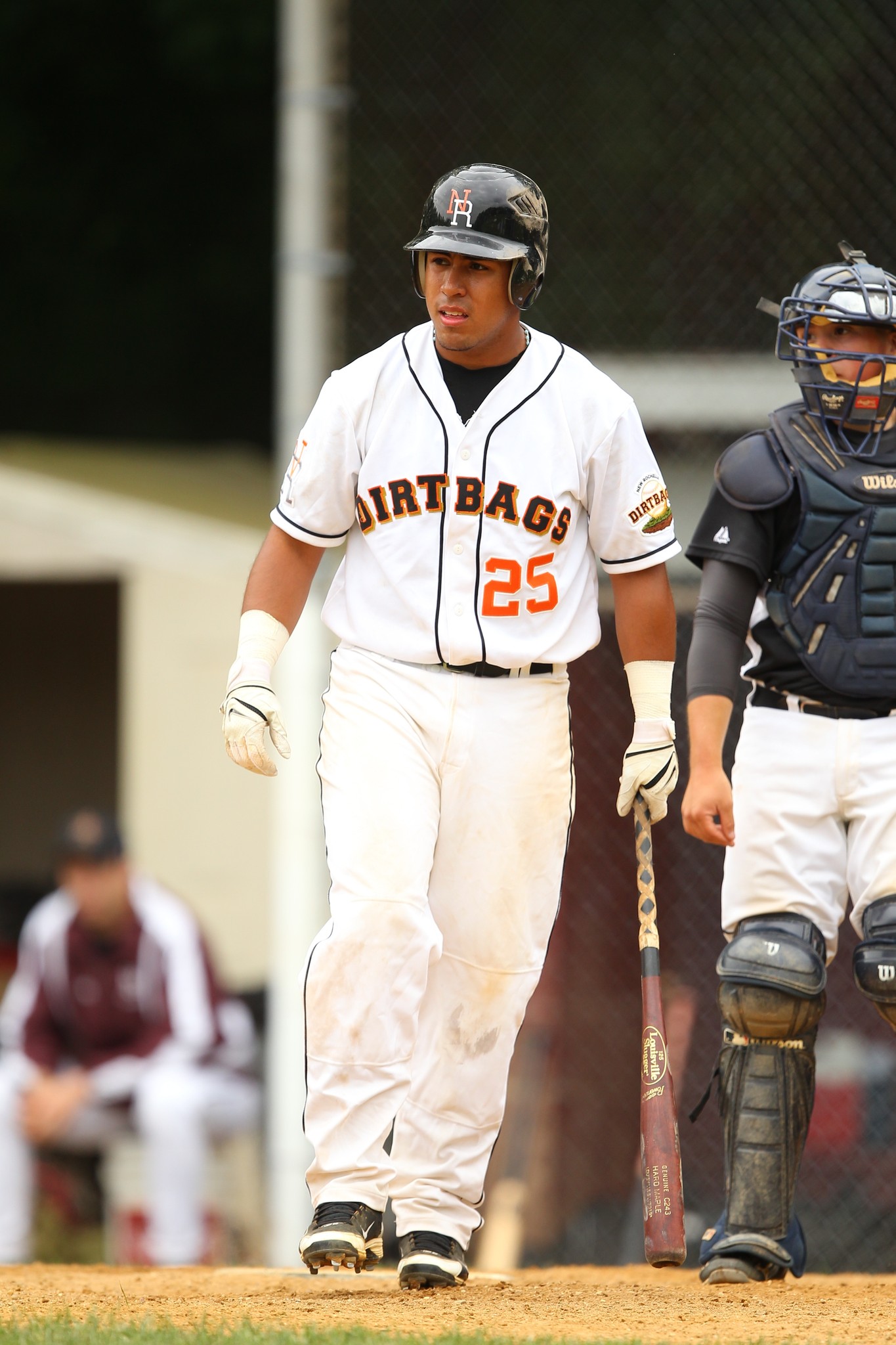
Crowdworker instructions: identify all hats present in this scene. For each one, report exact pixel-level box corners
[53,812,120,862]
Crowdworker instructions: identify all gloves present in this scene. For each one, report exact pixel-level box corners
[617,719,680,824]
[220,659,291,777]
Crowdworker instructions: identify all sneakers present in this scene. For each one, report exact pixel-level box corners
[700,1256,786,1283]
[397,1232,469,1290]
[298,1201,384,1274]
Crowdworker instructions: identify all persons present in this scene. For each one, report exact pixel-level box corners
[0,809,262,1272]
[200,163,683,1283]
[688,243,896,1285]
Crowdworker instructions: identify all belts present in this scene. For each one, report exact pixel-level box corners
[750,684,889,718]
[436,661,552,677]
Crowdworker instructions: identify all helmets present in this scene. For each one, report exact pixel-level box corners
[754,240,896,458]
[402,163,548,311]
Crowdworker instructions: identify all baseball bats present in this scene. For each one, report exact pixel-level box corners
[632,793,688,1269]
